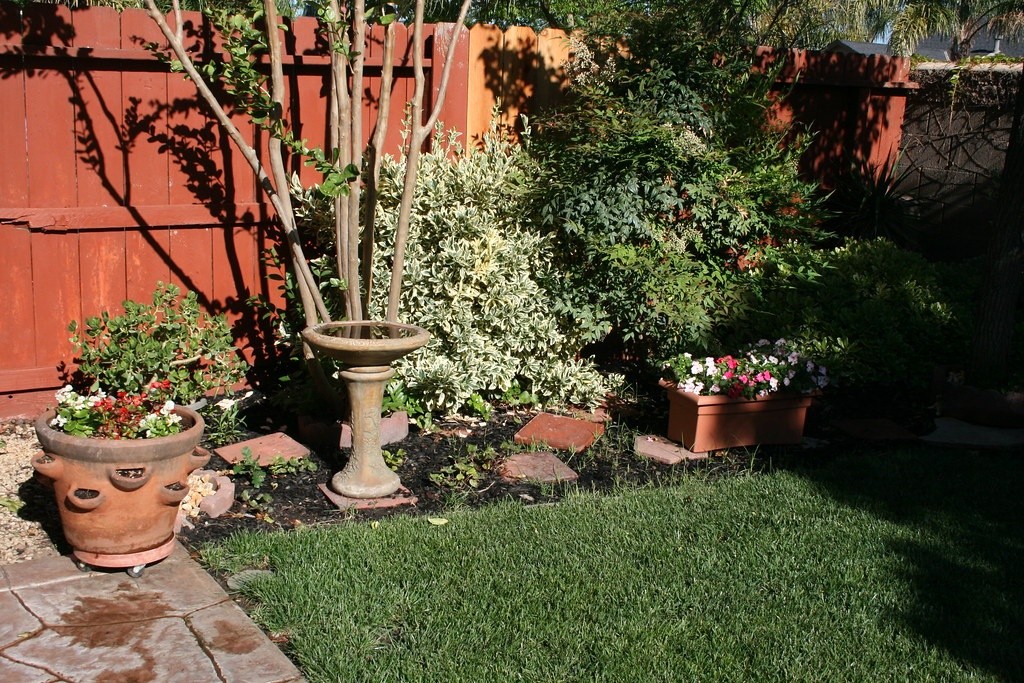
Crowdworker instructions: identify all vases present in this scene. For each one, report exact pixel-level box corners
[32,404,211,568]
[665,393,813,452]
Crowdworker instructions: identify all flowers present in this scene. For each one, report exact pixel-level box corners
[663,338,829,399]
[43,376,179,442]
